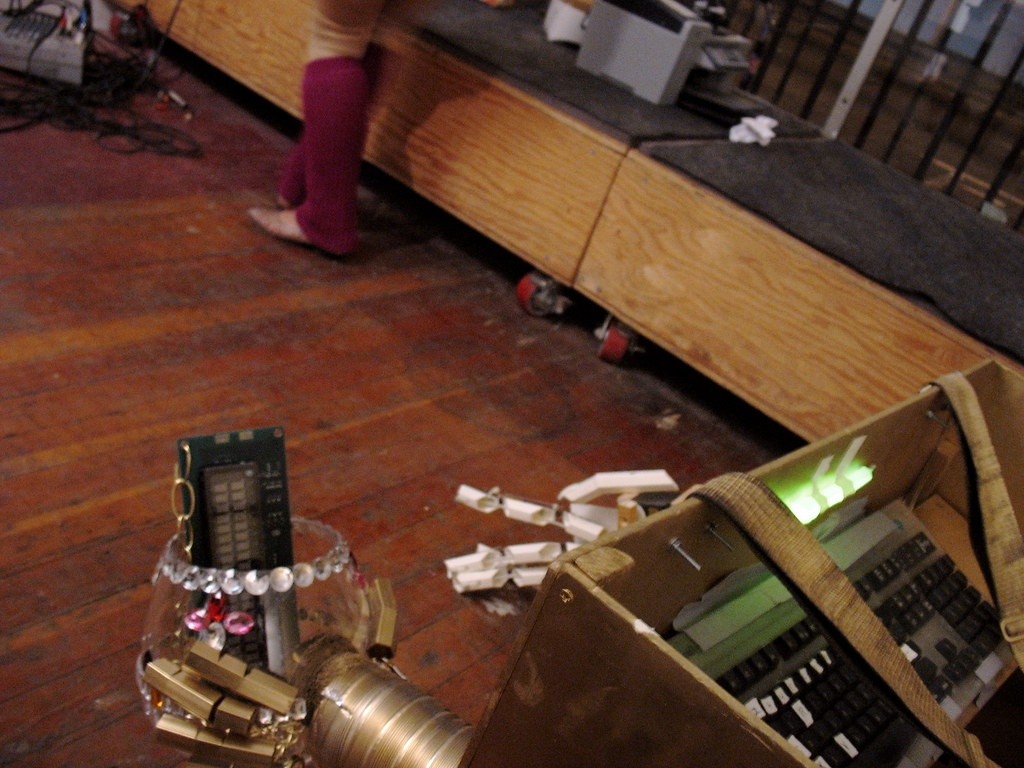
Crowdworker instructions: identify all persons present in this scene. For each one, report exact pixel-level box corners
[247,0,436,254]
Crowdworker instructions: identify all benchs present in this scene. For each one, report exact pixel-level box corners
[112,0,1024,445]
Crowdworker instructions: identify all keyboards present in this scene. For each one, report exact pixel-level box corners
[669,499,1012,768]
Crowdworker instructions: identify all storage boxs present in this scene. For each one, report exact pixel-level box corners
[461,359,1024,768]
